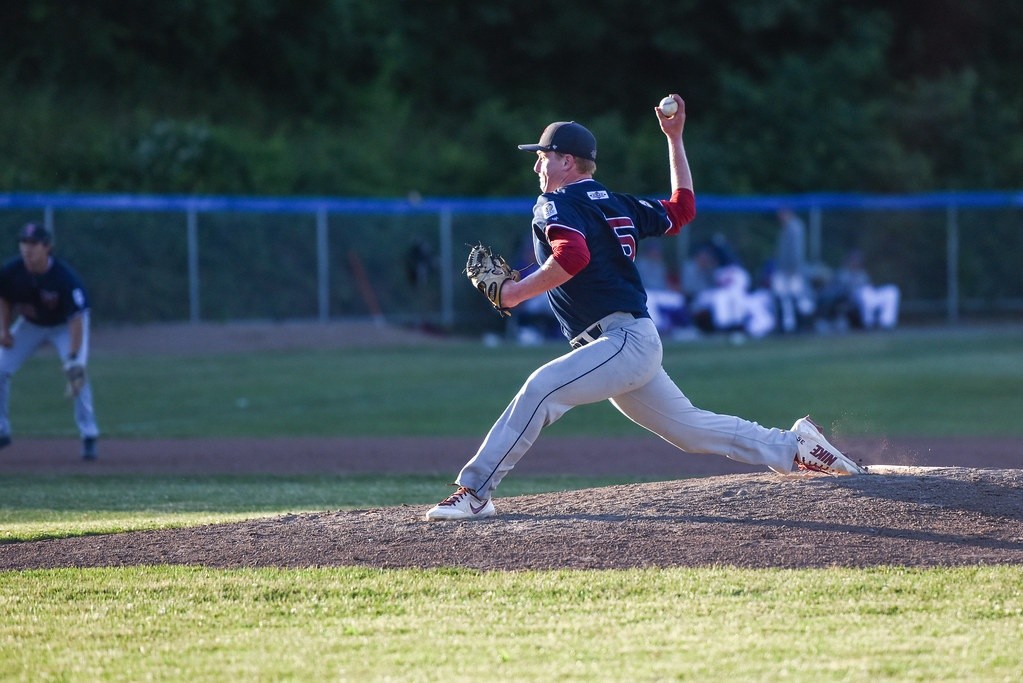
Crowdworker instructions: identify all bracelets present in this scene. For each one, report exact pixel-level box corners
[65,353,77,358]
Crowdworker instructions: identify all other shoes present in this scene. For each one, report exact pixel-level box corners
[82,440,95,458]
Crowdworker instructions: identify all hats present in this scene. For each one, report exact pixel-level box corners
[12,222,52,248]
[518,121,596,161]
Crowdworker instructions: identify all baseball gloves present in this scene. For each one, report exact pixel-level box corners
[466,241,521,317]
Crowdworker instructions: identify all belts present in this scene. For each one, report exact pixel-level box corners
[572,310,650,349]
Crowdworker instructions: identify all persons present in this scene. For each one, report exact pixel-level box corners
[474,239,560,348]
[0,223,102,462]
[644,216,900,346]
[424,92,866,522]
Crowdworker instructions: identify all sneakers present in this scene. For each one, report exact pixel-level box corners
[427,483,497,520]
[790,415,869,475]
